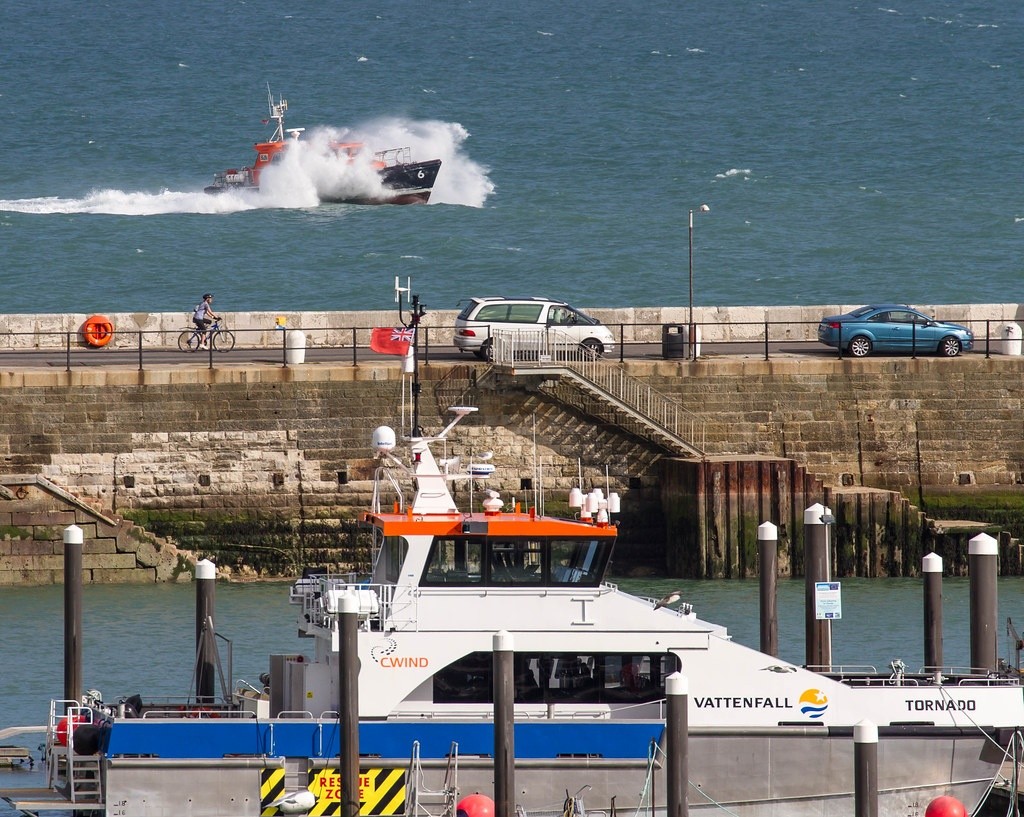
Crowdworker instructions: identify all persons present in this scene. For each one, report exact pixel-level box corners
[193,293,220,349]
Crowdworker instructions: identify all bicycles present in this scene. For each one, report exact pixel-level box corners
[178,318,236,353]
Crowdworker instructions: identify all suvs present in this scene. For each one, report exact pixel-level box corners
[456,294,616,363]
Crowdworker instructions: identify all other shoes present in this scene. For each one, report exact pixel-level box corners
[199,344,208,350]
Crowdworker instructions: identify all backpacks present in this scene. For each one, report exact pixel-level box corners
[194,304,200,312]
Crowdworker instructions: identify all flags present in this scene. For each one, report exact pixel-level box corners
[369,326,415,355]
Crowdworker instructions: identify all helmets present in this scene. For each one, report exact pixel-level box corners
[203,293,213,299]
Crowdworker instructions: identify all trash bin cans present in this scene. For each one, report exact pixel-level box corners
[661,324,684,360]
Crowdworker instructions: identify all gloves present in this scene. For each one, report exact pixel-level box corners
[216,316,222,321]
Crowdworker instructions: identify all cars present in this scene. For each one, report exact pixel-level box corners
[811,305,975,357]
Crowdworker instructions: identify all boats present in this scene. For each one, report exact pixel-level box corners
[1,292,1021,817]
[215,80,443,207]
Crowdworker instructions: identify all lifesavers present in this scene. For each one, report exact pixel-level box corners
[87,323,112,345]
